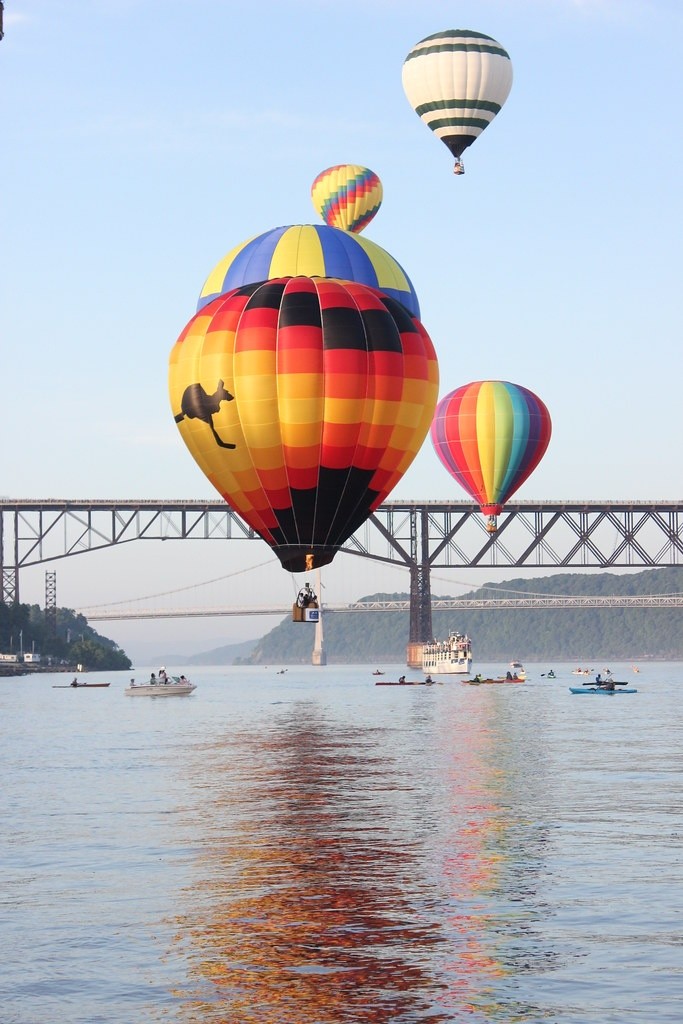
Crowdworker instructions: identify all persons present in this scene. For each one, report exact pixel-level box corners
[72,677,77,683]
[376,668,615,690]
[488,518,496,526]
[129,667,189,687]
[298,593,317,606]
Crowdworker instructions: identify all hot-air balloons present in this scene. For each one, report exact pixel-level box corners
[311,163,384,233]
[167,273,440,624]
[196,223,421,324]
[432,379,552,532]
[402,28,515,175]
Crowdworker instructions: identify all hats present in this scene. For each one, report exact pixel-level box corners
[160,666,166,671]
[608,678,614,683]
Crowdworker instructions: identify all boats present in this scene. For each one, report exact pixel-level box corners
[375,681,436,686]
[633,668,639,673]
[583,671,589,676]
[569,687,637,694]
[461,678,525,683]
[571,671,584,675]
[548,675,556,678]
[583,681,628,685]
[125,683,197,696]
[52,682,111,688]
[372,672,385,675]
